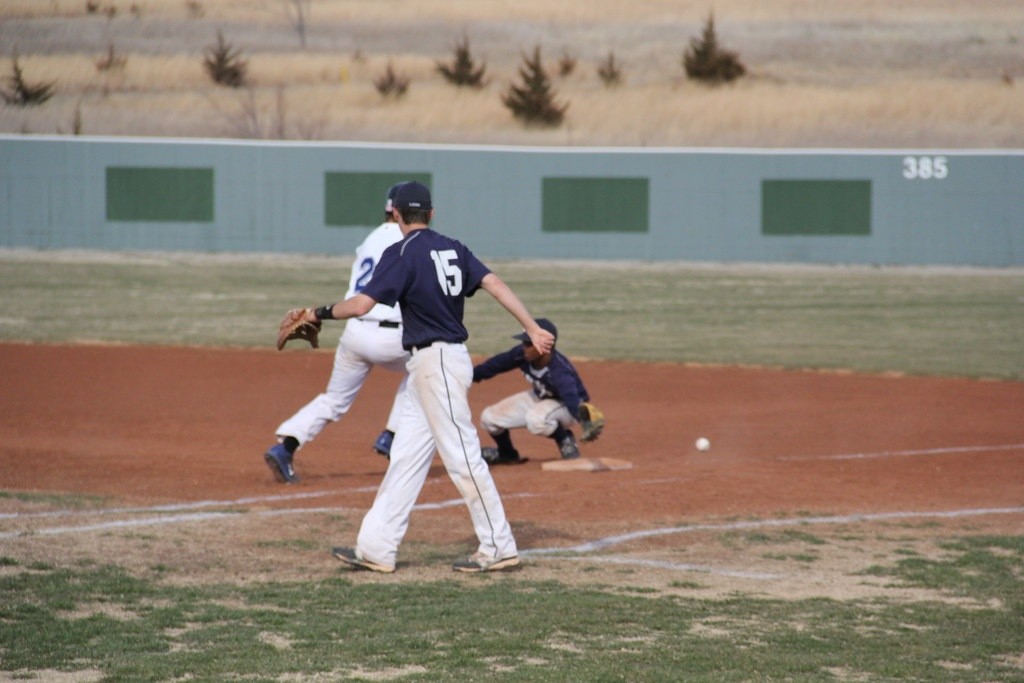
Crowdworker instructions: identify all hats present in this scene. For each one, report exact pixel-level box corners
[385,184,405,212]
[512,318,558,346]
[392,181,432,211]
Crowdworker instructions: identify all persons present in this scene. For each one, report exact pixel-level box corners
[263,179,413,486]
[276,181,554,574]
[473,318,605,466]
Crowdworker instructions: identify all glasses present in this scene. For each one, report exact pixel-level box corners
[521,341,532,348]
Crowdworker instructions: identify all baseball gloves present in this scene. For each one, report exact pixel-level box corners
[276,306,323,351]
[577,402,606,441]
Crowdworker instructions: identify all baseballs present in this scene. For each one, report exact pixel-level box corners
[695,437,710,451]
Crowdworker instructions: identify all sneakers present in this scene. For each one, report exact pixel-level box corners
[332,546,396,574]
[482,446,520,464]
[453,549,520,572]
[263,442,300,484]
[373,429,394,459]
[557,429,581,460]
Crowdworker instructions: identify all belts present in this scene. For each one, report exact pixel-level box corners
[410,340,432,356]
[379,320,399,329]
